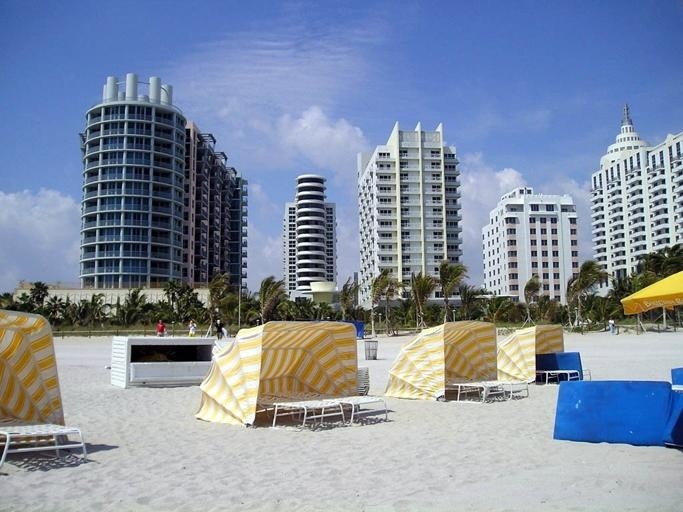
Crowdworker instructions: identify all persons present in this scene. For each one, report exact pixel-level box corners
[213,319,223,340]
[187,319,196,337]
[608,317,614,333]
[153,319,166,337]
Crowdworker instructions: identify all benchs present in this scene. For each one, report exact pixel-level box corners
[443,376,529,404]
[257,390,388,429]
[0,416,88,470]
[535,369,579,384]
